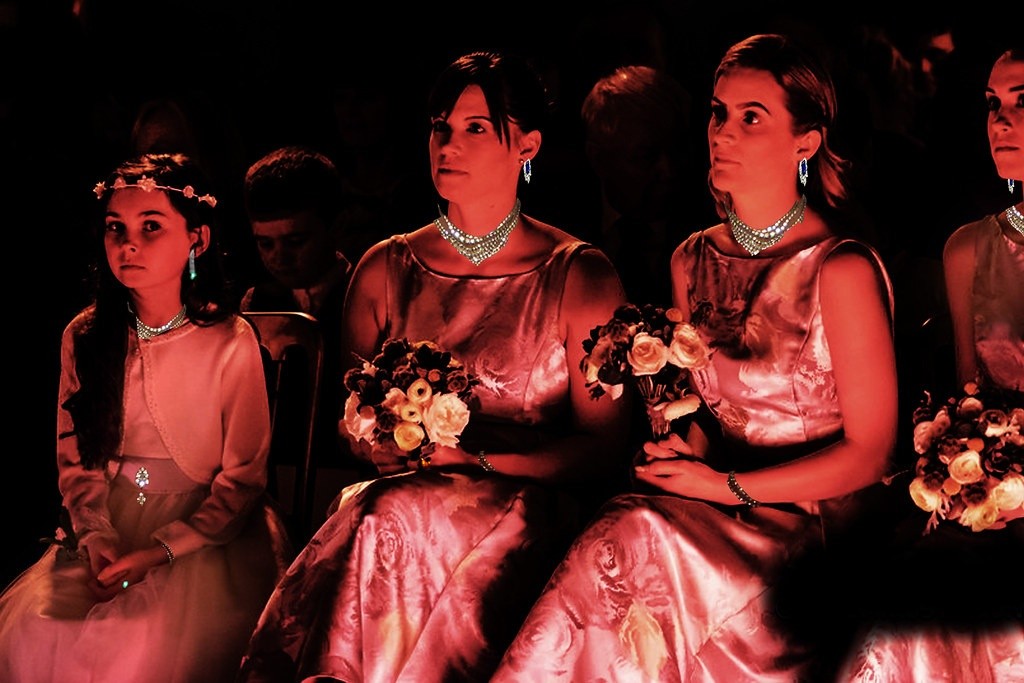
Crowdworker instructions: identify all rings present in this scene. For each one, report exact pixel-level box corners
[122,581,129,589]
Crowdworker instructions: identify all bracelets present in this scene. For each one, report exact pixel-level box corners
[161,542,175,563]
[727,471,761,512]
[477,451,495,473]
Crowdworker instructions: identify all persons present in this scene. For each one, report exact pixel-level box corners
[833,24,993,253]
[583,69,709,291]
[846,37,1024,683]
[237,149,351,356]
[244,54,633,683]
[487,29,900,683]
[3,154,273,683]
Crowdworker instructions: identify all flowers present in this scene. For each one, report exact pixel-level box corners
[908,382,1024,536]
[341,337,482,451]
[580,302,708,439]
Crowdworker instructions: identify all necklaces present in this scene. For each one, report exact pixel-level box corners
[1004,204,1024,238]
[433,196,524,267]
[726,195,811,257]
[137,303,189,340]
